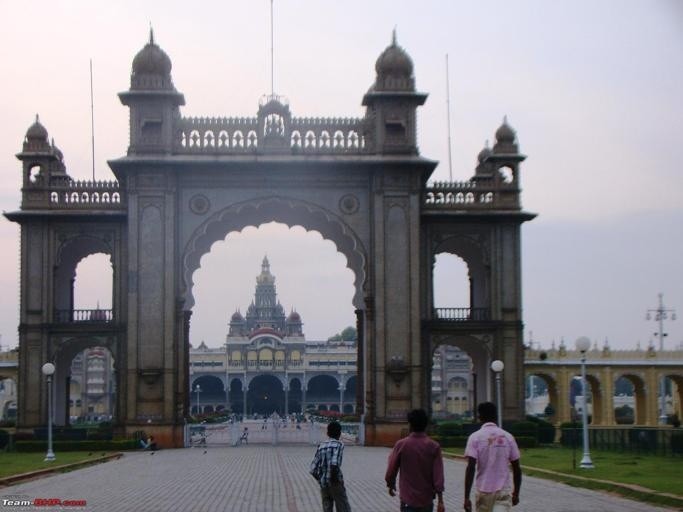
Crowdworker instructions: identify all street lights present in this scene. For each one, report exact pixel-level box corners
[194,384,204,415]
[492,359,505,429]
[646,291,677,426]
[576,335,596,469]
[42,363,57,463]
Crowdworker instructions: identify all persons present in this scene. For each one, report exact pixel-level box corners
[384,407,446,512]
[309,421,351,512]
[463,402,522,511]
[240,427,249,444]
[147,436,160,451]
[228,410,308,432]
